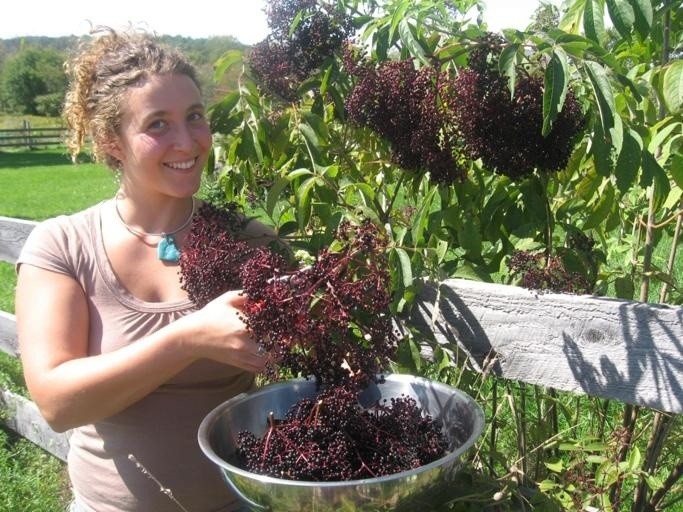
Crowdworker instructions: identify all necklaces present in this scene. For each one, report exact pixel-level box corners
[156,235,183,263]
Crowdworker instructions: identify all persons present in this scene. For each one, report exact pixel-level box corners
[13,17,325,512]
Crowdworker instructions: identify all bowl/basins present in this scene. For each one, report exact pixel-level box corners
[194,371,485,512]
